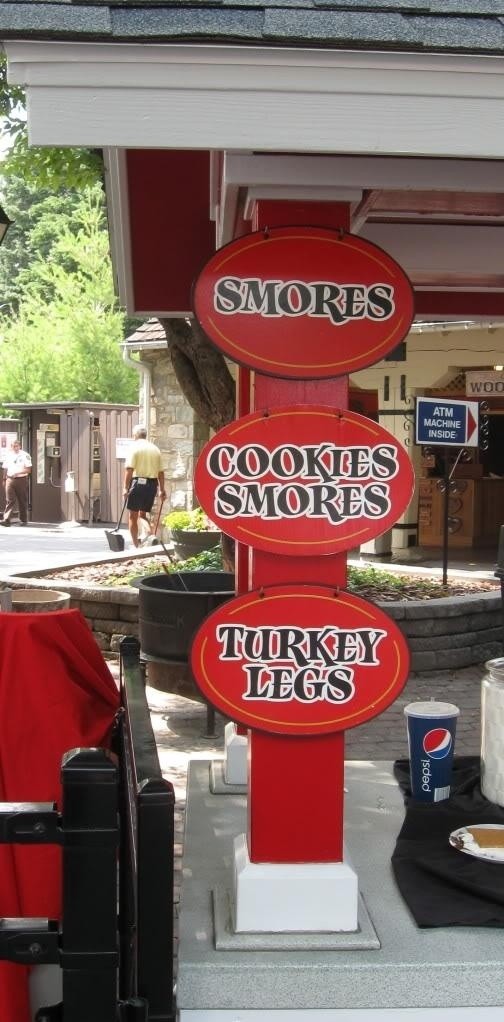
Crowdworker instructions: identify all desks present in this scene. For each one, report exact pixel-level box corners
[138,651,220,740]
[175,757,504,1021]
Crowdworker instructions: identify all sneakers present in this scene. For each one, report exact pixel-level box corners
[145,533,159,547]
[18,521,27,526]
[0,520,11,527]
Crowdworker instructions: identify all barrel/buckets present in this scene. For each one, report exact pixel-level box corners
[128,538,235,702]
[223,722,248,784]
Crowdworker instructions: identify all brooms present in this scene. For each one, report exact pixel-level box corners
[144,499,165,545]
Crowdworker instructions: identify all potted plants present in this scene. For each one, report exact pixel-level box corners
[163,507,223,564]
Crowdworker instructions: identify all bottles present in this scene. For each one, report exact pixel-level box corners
[479,655,503,809]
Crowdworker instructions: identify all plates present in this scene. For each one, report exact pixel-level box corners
[446,824,503,866]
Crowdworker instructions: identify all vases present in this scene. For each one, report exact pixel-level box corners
[126,567,235,662]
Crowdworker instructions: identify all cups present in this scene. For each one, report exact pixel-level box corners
[404,703,459,806]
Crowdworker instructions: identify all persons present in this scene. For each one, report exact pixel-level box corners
[0,440,33,527]
[122,423,167,547]
